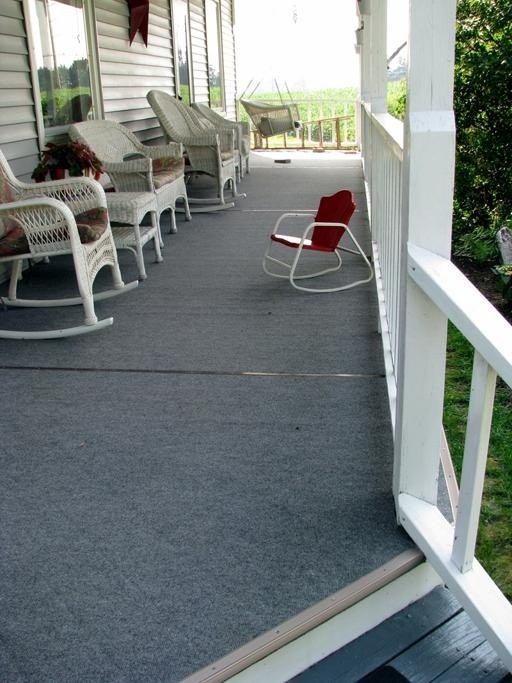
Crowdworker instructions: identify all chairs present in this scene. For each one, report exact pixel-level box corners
[261,189,374,293]
[0,155,163,342]
[0,90,250,234]
[239,99,302,138]
